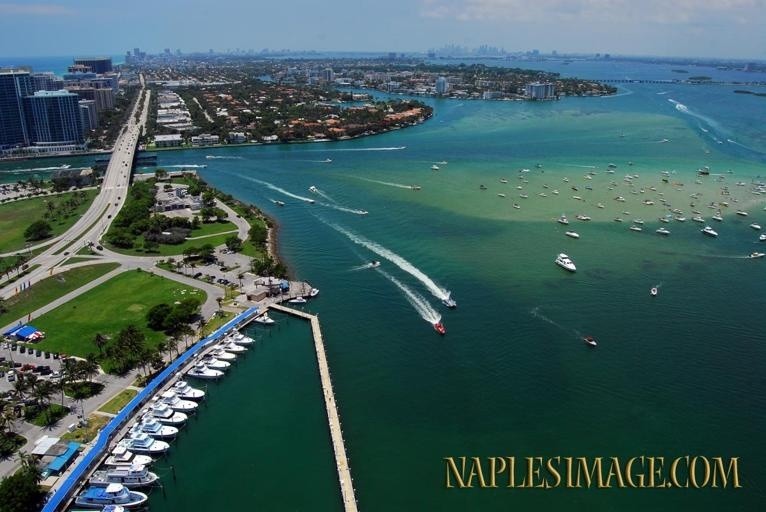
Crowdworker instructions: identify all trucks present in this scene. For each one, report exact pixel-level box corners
[22,264,29,270]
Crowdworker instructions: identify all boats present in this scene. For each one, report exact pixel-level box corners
[583,336,598,347]
[273,185,318,207]
[253,312,275,324]
[410,182,422,191]
[368,261,380,267]
[479,160,766,297]
[433,298,456,336]
[355,209,369,216]
[198,145,448,170]
[288,288,320,304]
[75,326,256,512]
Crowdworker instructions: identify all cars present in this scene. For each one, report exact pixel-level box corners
[0,342,68,418]
[96,245,103,250]
[193,247,243,289]
[108,133,137,218]
[64,251,70,256]
